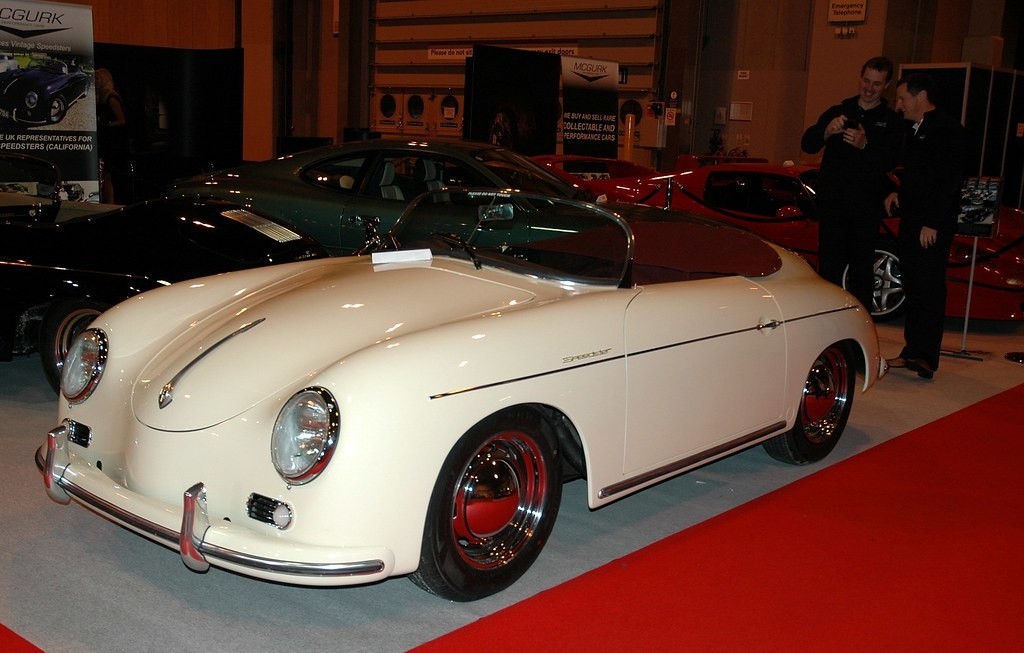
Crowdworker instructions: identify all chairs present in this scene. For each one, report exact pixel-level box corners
[365,160,405,202]
[411,156,449,201]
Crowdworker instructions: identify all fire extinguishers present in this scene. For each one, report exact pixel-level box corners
[714,144,749,165]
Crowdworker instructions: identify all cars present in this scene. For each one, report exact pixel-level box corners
[540,151,1023,329]
[151,135,576,258]
[34,189,892,604]
[1,151,328,392]
[0,54,93,126]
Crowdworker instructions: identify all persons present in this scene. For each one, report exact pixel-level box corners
[488,110,514,149]
[15,184,28,194]
[2,184,14,193]
[57,180,69,201]
[94,68,129,205]
[800,55,904,316]
[884,72,971,379]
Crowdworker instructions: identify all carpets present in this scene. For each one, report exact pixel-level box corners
[406,381,1023,653]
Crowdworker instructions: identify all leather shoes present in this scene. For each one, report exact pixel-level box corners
[882,356,935,379]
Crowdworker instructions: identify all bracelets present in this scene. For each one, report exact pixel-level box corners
[108,120,112,126]
[859,136,868,150]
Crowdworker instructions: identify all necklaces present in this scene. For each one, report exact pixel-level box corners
[859,96,881,110]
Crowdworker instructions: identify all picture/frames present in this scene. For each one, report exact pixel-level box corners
[730,101,752,121]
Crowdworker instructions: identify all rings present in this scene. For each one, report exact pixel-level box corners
[851,137,856,142]
